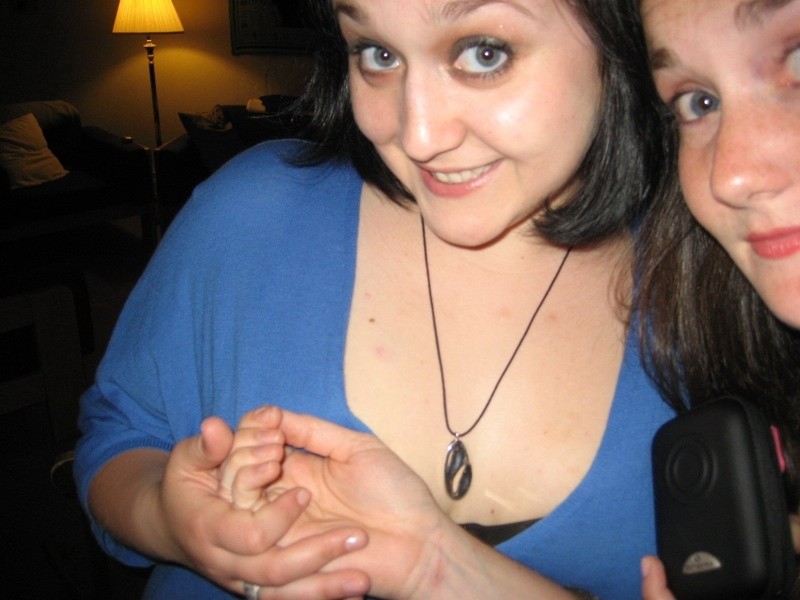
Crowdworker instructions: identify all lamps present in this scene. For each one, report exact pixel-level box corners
[112,0,185,147]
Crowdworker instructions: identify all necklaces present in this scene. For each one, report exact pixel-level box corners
[421,214,573,500]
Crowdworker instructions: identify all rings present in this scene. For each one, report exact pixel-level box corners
[244,584,260,600]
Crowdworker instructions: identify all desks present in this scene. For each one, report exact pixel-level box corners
[0,235,94,357]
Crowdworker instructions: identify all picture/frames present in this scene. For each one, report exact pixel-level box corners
[228,0,323,56]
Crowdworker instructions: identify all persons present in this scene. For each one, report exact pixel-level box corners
[71,0,687,600]
[219,0,800,600]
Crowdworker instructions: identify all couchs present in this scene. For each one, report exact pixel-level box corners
[0,99,152,281]
[155,95,315,237]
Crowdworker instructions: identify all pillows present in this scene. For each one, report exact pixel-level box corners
[176,105,240,176]
[0,111,70,189]
